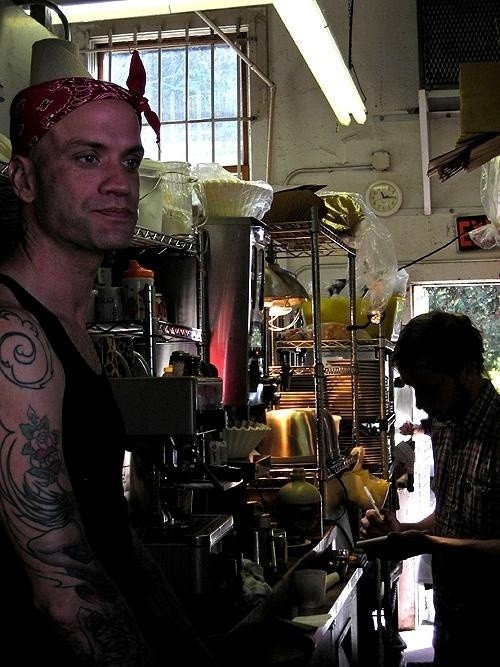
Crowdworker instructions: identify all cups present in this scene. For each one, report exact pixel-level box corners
[258,529,349,608]
[99,286,125,320]
[161,160,207,233]
[173,486,194,516]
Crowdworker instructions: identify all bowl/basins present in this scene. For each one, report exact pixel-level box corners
[192,181,272,219]
[265,409,337,464]
[221,421,269,459]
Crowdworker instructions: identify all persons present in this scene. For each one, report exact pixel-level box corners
[358,309,499,667]
[1,79,213,667]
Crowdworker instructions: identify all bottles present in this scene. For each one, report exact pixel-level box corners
[210,436,229,466]
[121,260,155,319]
[274,467,324,545]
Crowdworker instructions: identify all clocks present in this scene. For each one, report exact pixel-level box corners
[365,180,403,218]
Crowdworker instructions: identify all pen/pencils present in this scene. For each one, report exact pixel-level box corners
[364,486,384,521]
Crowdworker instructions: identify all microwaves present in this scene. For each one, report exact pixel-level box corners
[109,377,223,434]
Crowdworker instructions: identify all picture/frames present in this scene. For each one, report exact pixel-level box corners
[454,214,493,254]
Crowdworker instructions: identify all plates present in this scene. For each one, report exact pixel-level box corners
[281,356,386,465]
